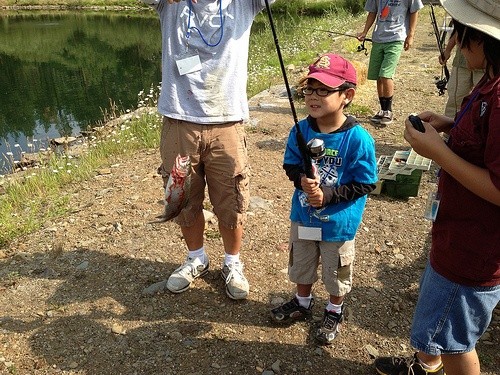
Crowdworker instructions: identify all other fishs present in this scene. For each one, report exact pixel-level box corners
[147,153,192,224]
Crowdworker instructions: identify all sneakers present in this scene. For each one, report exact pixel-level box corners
[166,256,209,293]
[316,303,344,343]
[374,352,444,375]
[371,109,384,122]
[380,110,393,125]
[221,262,249,300]
[270,298,315,323]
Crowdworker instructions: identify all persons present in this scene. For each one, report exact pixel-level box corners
[270,53,379,344]
[374,0,500,375]
[156,0,277,299]
[355,0,425,125]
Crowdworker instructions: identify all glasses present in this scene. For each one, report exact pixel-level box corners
[301,87,346,96]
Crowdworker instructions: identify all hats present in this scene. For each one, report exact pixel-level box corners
[299,53,357,88]
[440,0,500,40]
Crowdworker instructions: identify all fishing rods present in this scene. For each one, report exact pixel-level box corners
[280,26,373,57]
[264,0,326,186]
[427,2,450,95]
